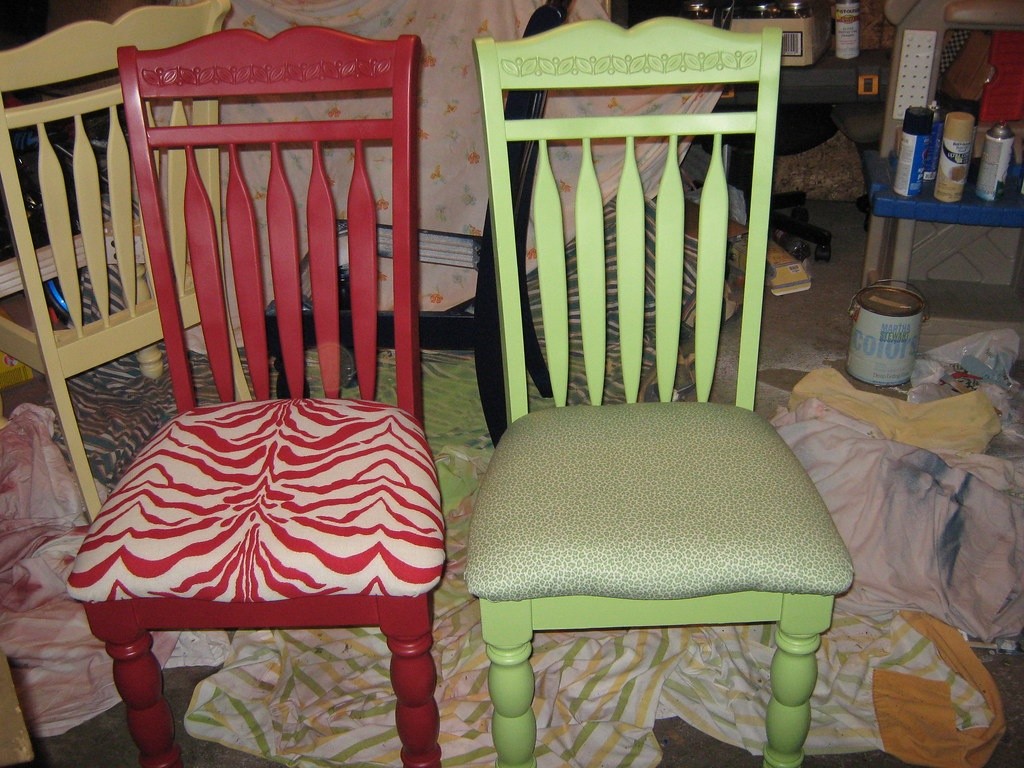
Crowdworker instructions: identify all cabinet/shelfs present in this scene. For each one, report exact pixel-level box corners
[858,0,1024,352]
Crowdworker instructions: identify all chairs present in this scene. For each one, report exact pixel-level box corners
[0,0,250,529]
[264,0,573,449]
[465,16,856,768]
[68,25,449,768]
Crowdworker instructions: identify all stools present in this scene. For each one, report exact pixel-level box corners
[693,103,842,263]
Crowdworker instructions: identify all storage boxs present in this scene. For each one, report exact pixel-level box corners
[676,0,833,66]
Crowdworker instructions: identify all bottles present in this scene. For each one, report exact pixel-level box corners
[922,101,942,191]
[976,121,1016,202]
[835,0,860,59]
[749,0,809,18]
[892,106,935,198]
[934,111,976,202]
[687,3,705,19]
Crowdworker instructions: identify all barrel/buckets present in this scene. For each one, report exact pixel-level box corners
[844,278,931,386]
